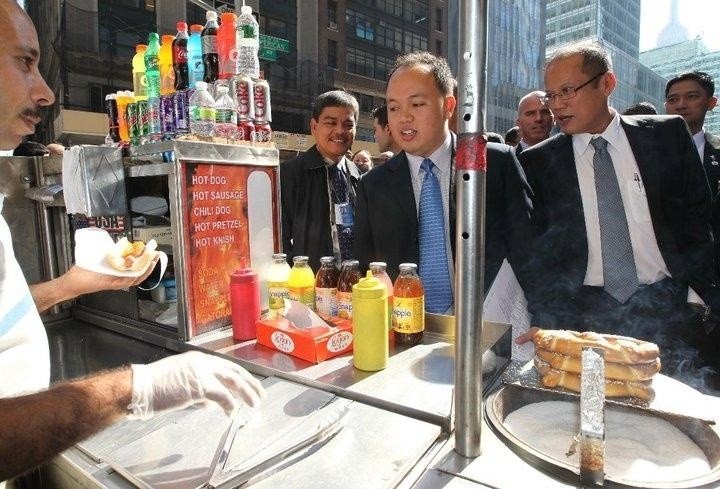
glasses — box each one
[539,73,601,110]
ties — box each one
[419,159,453,314]
[329,164,355,260]
[589,137,638,305]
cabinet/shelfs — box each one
[34,137,291,344]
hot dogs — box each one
[106,236,158,272]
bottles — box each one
[367,261,399,339]
[228,254,260,341]
[131,5,261,100]
[184,78,236,139]
[265,252,362,322]
[103,89,134,148]
[351,270,389,372]
[394,263,427,346]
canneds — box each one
[125,79,273,162]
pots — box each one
[485,381,720,487]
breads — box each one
[534,329,663,402]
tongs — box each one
[200,401,354,489]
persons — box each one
[0,0,268,485]
[14,136,65,156]
[504,38,720,373]
[281,90,362,273]
[355,50,536,319]
[345,105,403,171]
